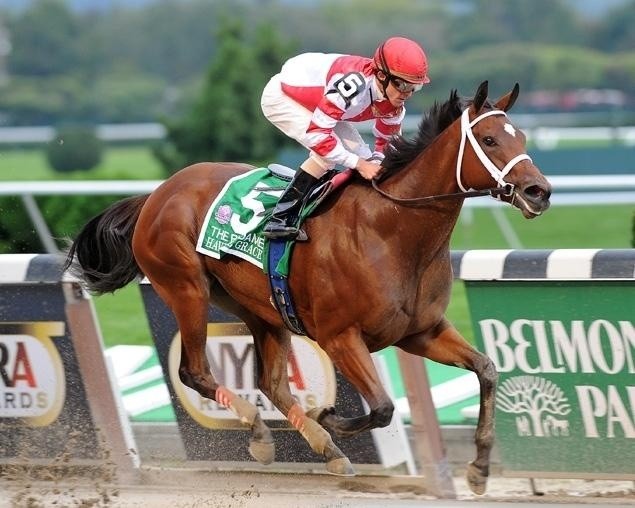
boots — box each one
[261,165,321,240]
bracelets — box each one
[342,153,360,170]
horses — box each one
[54,79,553,495]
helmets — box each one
[372,35,431,84]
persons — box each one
[261,37,432,244]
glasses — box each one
[389,76,423,94]
